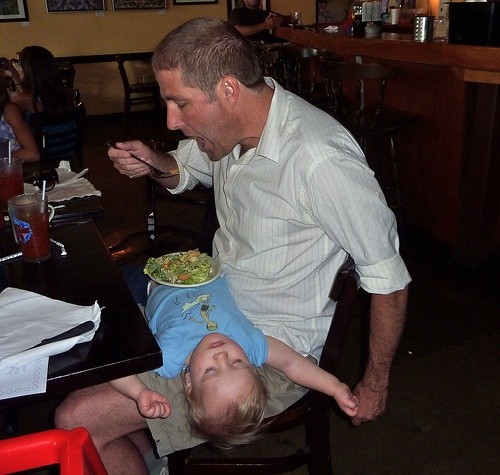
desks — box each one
[0,160,105,228]
[0,215,163,409]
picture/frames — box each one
[227,0,270,22]
[112,0,166,11]
[45,0,105,13]
[0,0,30,22]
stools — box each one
[253,41,421,226]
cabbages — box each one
[143,249,214,285]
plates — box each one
[148,252,220,288]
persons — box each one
[108,264,359,450]
[52,17,412,475]
[231,0,288,51]
[0,46,79,168]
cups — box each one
[290,8,301,24]
[391,9,400,25]
[0,157,24,213]
[7,192,52,264]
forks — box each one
[106,141,179,178]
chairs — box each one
[116,55,162,122]
[31,70,86,169]
[168,257,361,475]
[109,139,219,267]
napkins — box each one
[44,160,102,202]
[0,287,105,372]
